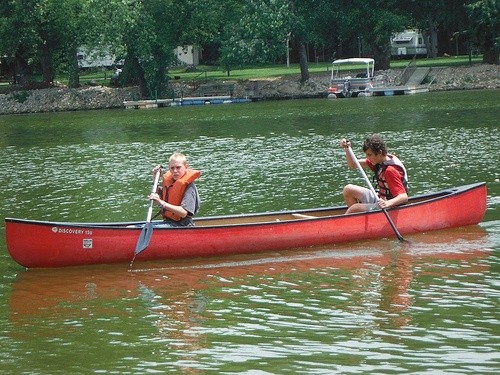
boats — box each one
[5,181,486,268]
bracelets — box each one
[159,201,166,209]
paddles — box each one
[135,164,163,254]
[345,139,409,243]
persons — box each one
[111,65,123,79]
[123,154,203,228]
[338,135,410,215]
